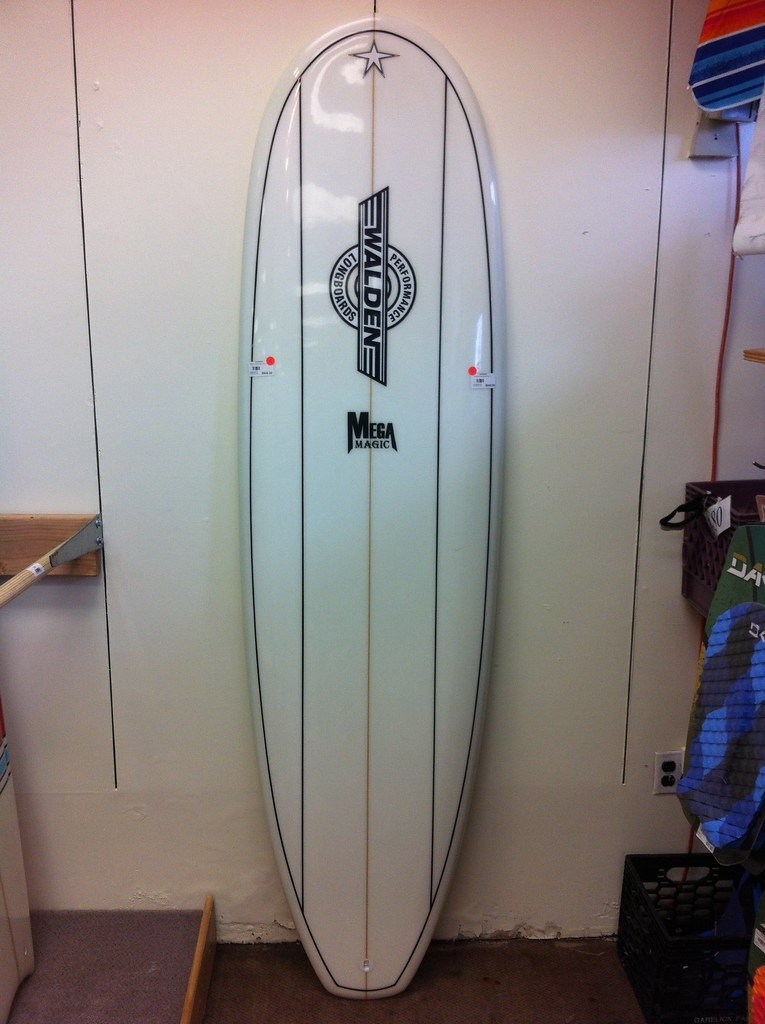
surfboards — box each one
[236,16,511,1000]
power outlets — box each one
[653,750,684,794]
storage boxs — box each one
[682,479,765,619]
[617,854,758,1024]
